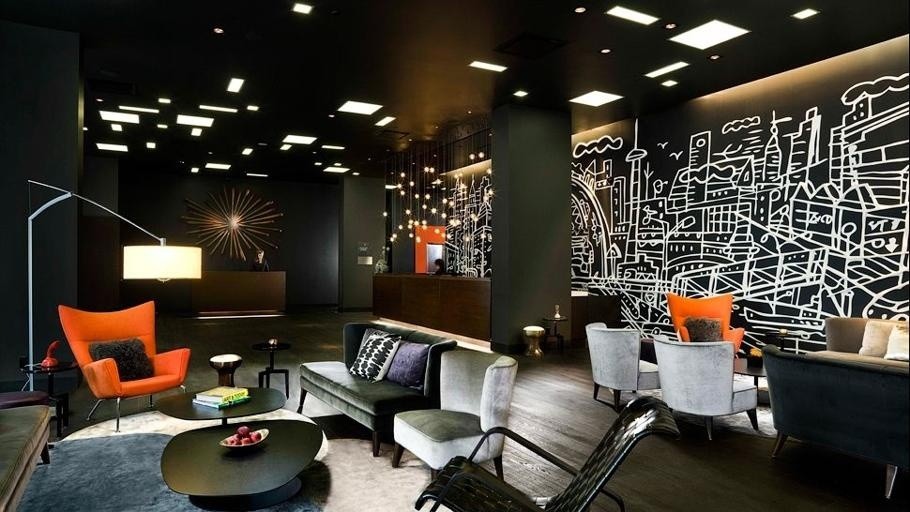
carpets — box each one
[608,372,804,444]
[17,432,331,512]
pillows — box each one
[385,340,432,391]
[884,324,909,361]
[88,337,155,382]
[349,327,402,384]
[684,316,722,342]
[858,319,904,357]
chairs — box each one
[415,395,682,512]
[585,322,661,412]
[653,334,758,441]
[667,292,745,358]
[57,300,192,433]
[392,349,519,481]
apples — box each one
[226,426,262,445]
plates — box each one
[219,427,270,452]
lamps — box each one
[20,178,203,391]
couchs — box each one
[805,316,909,374]
[0,391,52,512]
[297,322,457,457]
[761,345,910,499]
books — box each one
[196,386,248,403]
[192,396,251,409]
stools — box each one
[542,315,568,349]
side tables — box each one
[18,360,79,437]
[251,341,290,401]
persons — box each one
[254,250,269,272]
[433,259,445,275]
